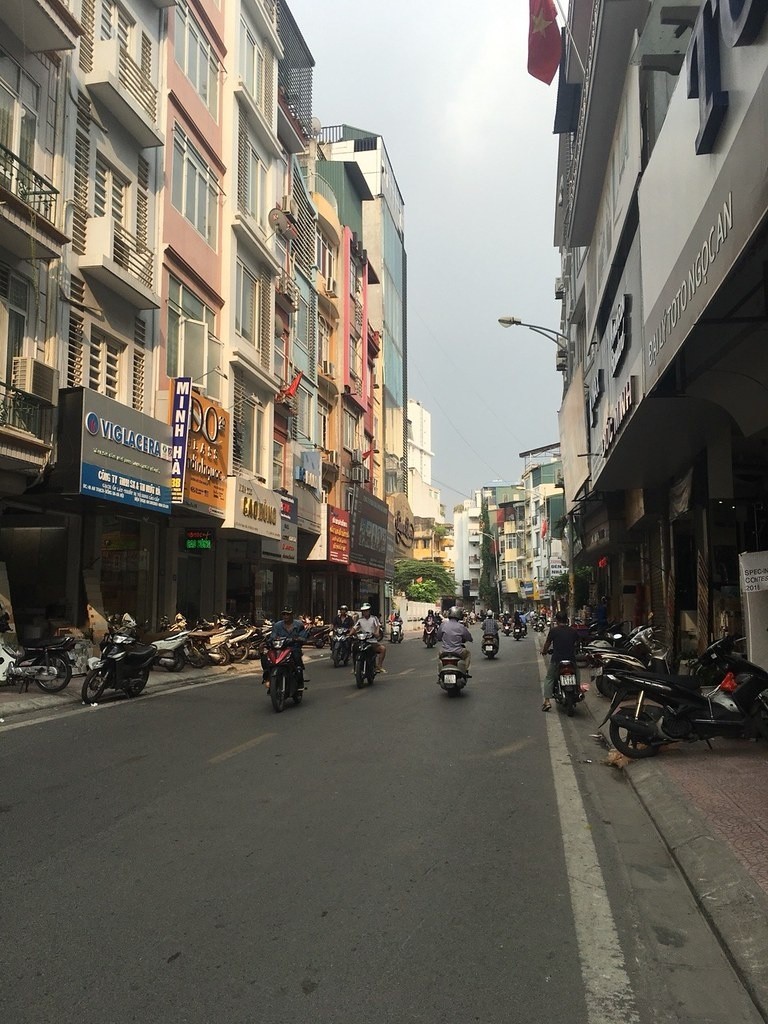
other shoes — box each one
[297,687,304,691]
[375,668,386,673]
[466,674,472,678]
[542,703,551,710]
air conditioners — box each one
[374,374,379,387]
[328,450,339,464]
[281,194,298,222]
[325,277,340,298]
[351,449,362,464]
[556,338,568,357]
[322,360,336,380]
[526,517,531,527]
[359,469,362,483]
[364,469,370,483]
[352,468,359,481]
[554,277,563,299]
[556,356,567,371]
[10,357,61,410]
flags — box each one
[285,372,302,397]
[363,450,372,461]
[541,521,547,538]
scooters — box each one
[420,616,443,649]
[115,614,276,672]
[479,626,502,659]
[261,633,310,712]
[436,639,474,698]
[304,621,385,689]
[458,618,477,628]
[499,613,547,641]
[386,617,405,643]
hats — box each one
[280,605,293,613]
[361,602,371,611]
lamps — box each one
[248,475,266,485]
[192,365,228,382]
[300,443,317,448]
[498,316,568,350]
[296,437,310,442]
[306,447,324,451]
[312,450,330,455]
[224,392,263,410]
[271,486,289,496]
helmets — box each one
[340,605,348,612]
[487,609,493,616]
[448,606,462,619]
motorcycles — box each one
[571,604,768,758]
[0,601,76,692]
[81,615,160,704]
[540,648,587,716]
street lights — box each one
[475,530,501,614]
[514,486,554,628]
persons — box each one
[436,606,473,670]
[540,611,581,711]
[530,606,547,622]
[461,608,476,622]
[391,613,403,629]
[330,605,359,654]
[260,606,308,687]
[481,609,499,645]
[423,609,449,637]
[499,610,531,634]
[596,595,610,639]
[347,603,388,673]
